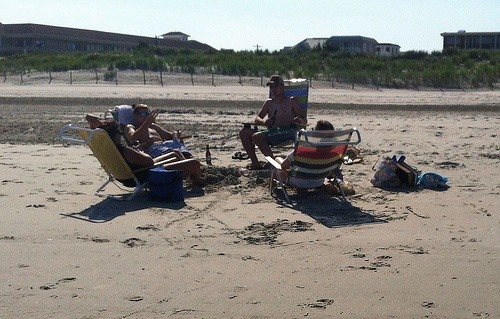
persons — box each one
[270,120,341,189]
[238,76,308,170]
[86,103,212,188]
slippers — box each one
[342,154,359,161]
[239,153,250,160]
[343,158,362,164]
[232,151,242,159]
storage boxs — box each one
[149,168,184,202]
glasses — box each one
[269,85,281,88]
[112,128,123,134]
[136,111,149,115]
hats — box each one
[97,118,120,130]
[266,75,284,86]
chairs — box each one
[58,105,190,202]
[265,126,362,205]
[242,79,308,157]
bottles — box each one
[206,143,211,163]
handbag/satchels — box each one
[369,155,407,188]
